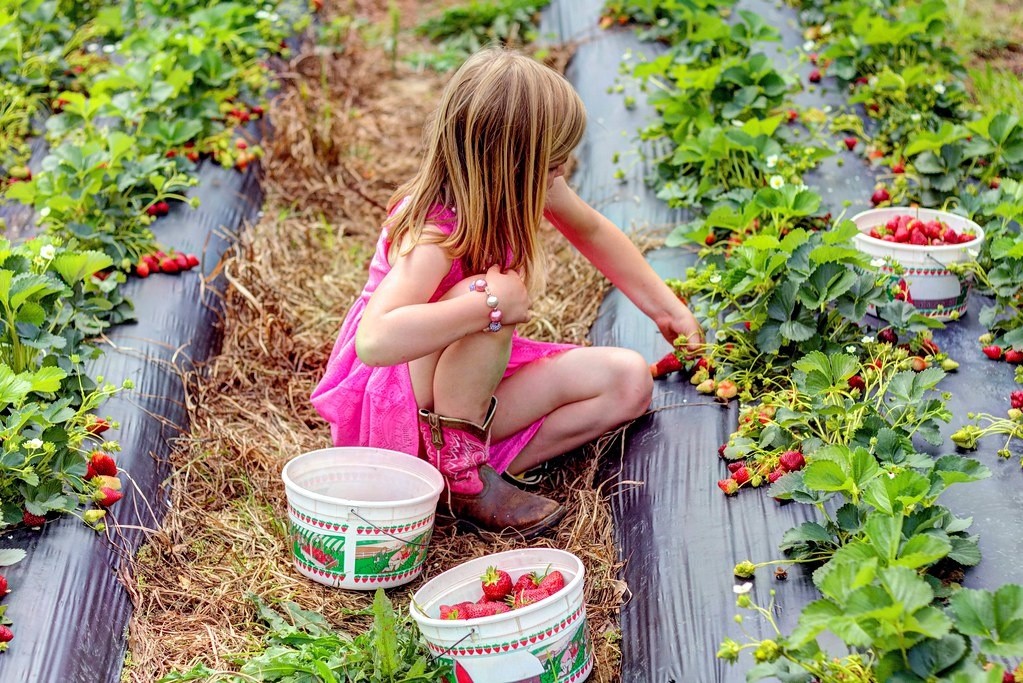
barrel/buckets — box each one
[280,446,446,592]
[847,206,985,322]
[409,547,594,683]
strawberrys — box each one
[0,0,324,642]
[600,14,1023,683]
[439,563,565,621]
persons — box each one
[308,49,706,538]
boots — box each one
[419,393,571,541]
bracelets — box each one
[468,277,504,333]
